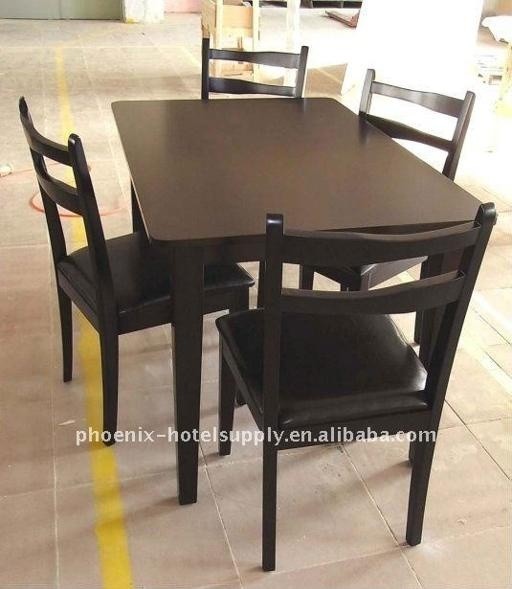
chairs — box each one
[19,36,496,572]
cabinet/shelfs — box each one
[201,0,258,82]
